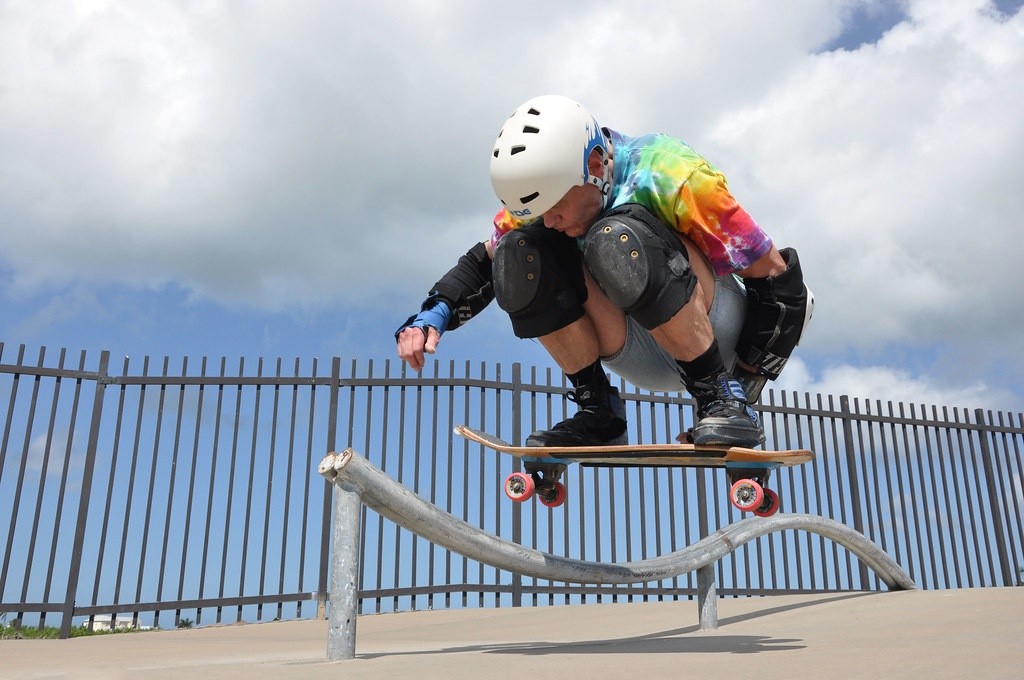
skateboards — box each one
[452,421,815,518]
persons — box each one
[393,95,808,447]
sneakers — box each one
[677,368,766,447]
[525,387,630,447]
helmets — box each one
[490,95,609,220]
